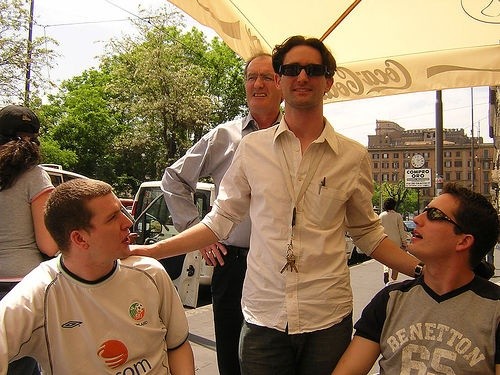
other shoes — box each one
[384,272,389,284]
[391,269,398,280]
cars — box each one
[403,220,416,232]
[38,162,217,309]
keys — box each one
[279,253,298,274]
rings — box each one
[207,251,211,254]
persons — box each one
[0,179,194,375]
[378,198,408,285]
[331,181,500,375]
[161,54,284,375]
[0,105,60,375]
[121,35,425,375]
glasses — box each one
[279,64,326,77]
[421,206,467,234]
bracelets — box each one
[414,262,425,279]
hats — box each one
[0,105,40,137]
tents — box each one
[164,0,500,105]
